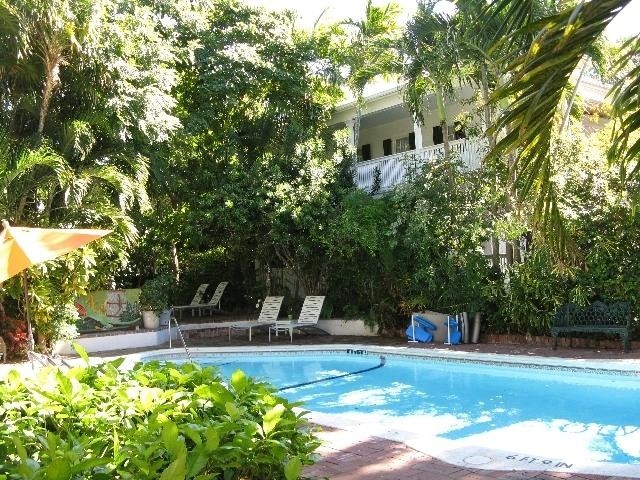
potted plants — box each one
[138,280,165,330]
[159,277,177,327]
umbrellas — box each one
[1,219,114,373]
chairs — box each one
[551,303,632,353]
[170,283,209,317]
[268,296,330,344]
[170,281,230,321]
[229,296,285,342]
